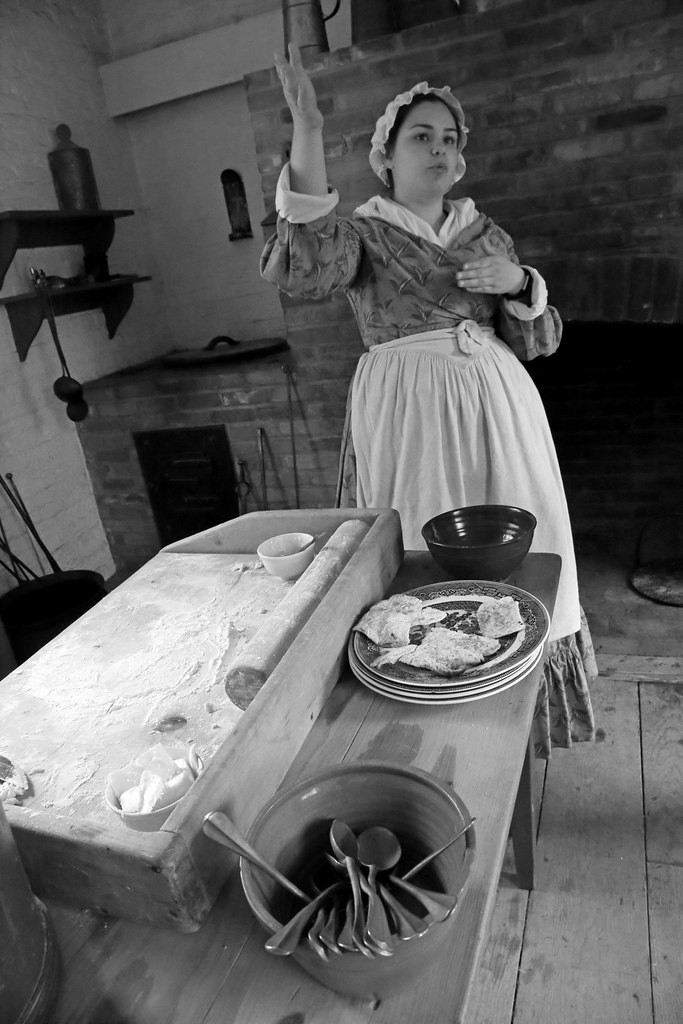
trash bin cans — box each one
[0,569,107,663]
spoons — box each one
[329,818,375,962]
[357,824,403,950]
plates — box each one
[347,580,550,704]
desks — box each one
[36,547,564,1024]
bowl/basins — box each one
[256,533,315,579]
[104,748,203,831]
[421,504,537,583]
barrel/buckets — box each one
[0,570,110,666]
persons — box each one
[256,40,597,754]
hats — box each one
[369,81,470,188]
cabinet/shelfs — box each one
[0,208,152,362]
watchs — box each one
[504,266,532,301]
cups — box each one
[239,759,476,1001]
